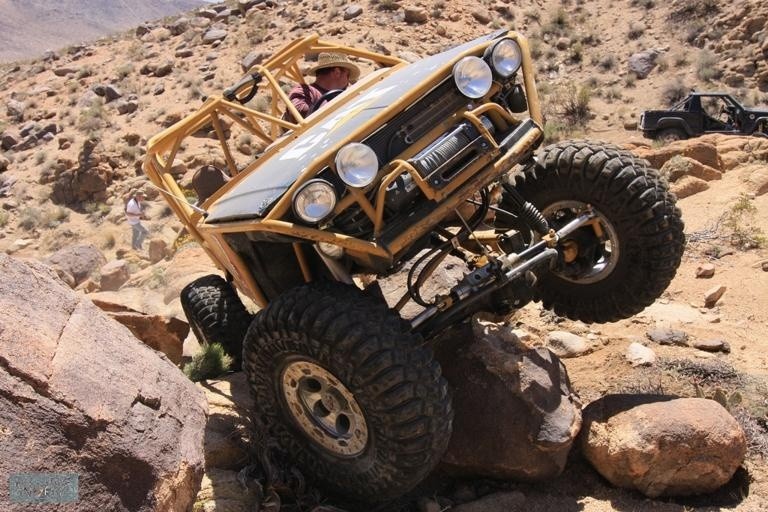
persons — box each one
[284,50,359,131]
[124,192,149,251]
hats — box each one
[133,189,147,198]
[307,52,360,80]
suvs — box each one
[141,30,688,509]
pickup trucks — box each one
[637,90,768,142]
